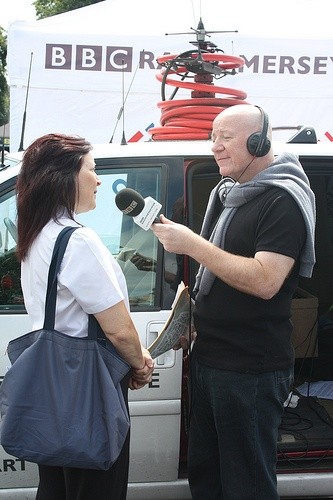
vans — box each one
[1,19,333,500]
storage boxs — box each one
[289,287,319,359]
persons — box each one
[152,104,308,500]
[15,133,152,499]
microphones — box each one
[115,188,164,231]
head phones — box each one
[247,105,271,157]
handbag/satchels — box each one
[0,225,133,471]
